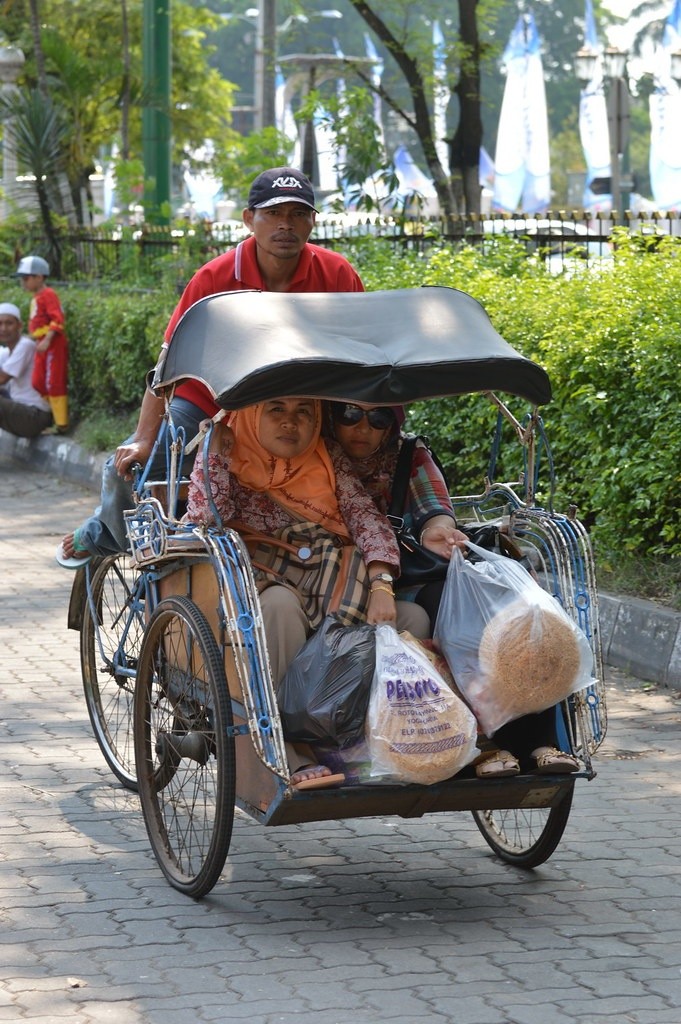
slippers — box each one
[54,527,92,568]
[292,763,345,789]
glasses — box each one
[329,402,395,431]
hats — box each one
[10,255,50,278]
[0,302,20,318]
[248,167,319,214]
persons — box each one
[0,255,69,439]
[186,398,582,791]
[55,167,365,569]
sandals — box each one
[478,749,520,776]
[527,747,580,777]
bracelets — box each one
[370,587,396,597]
[419,527,430,546]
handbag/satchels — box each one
[392,526,497,589]
[432,540,600,740]
[276,610,481,787]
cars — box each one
[212,211,608,271]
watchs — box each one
[369,572,394,586]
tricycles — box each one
[66,283,604,897]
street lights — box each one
[572,46,632,224]
